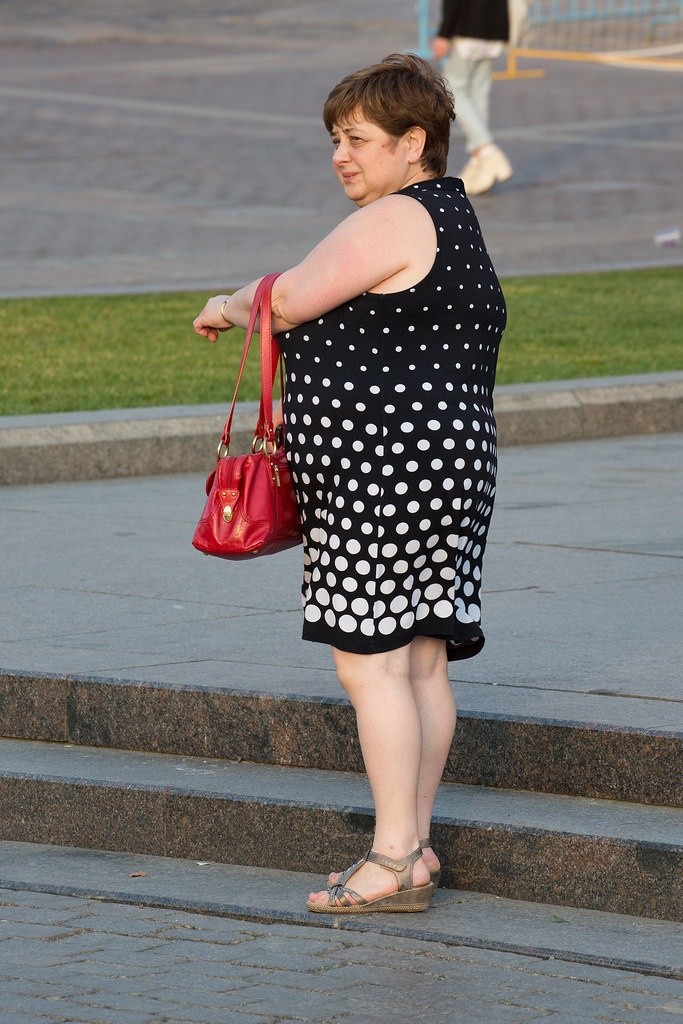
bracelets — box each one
[220,295,235,328]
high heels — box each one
[460,147,513,195]
[326,837,442,896]
[305,847,435,913]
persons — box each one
[426,0,516,198]
[193,52,507,914]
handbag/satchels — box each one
[191,271,303,559]
